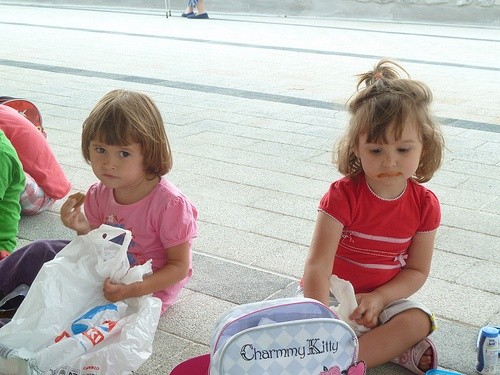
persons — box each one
[0,88,198,331]
[0,128,26,259]
[181,0,209,19]
[0,95,73,213]
[302,59,442,375]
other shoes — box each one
[187,13,209,19]
[182,11,195,17]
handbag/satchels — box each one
[207,298,367,375]
[0,224,162,375]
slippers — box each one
[391,338,438,375]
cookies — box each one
[68,192,86,208]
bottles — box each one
[476,326,500,375]
